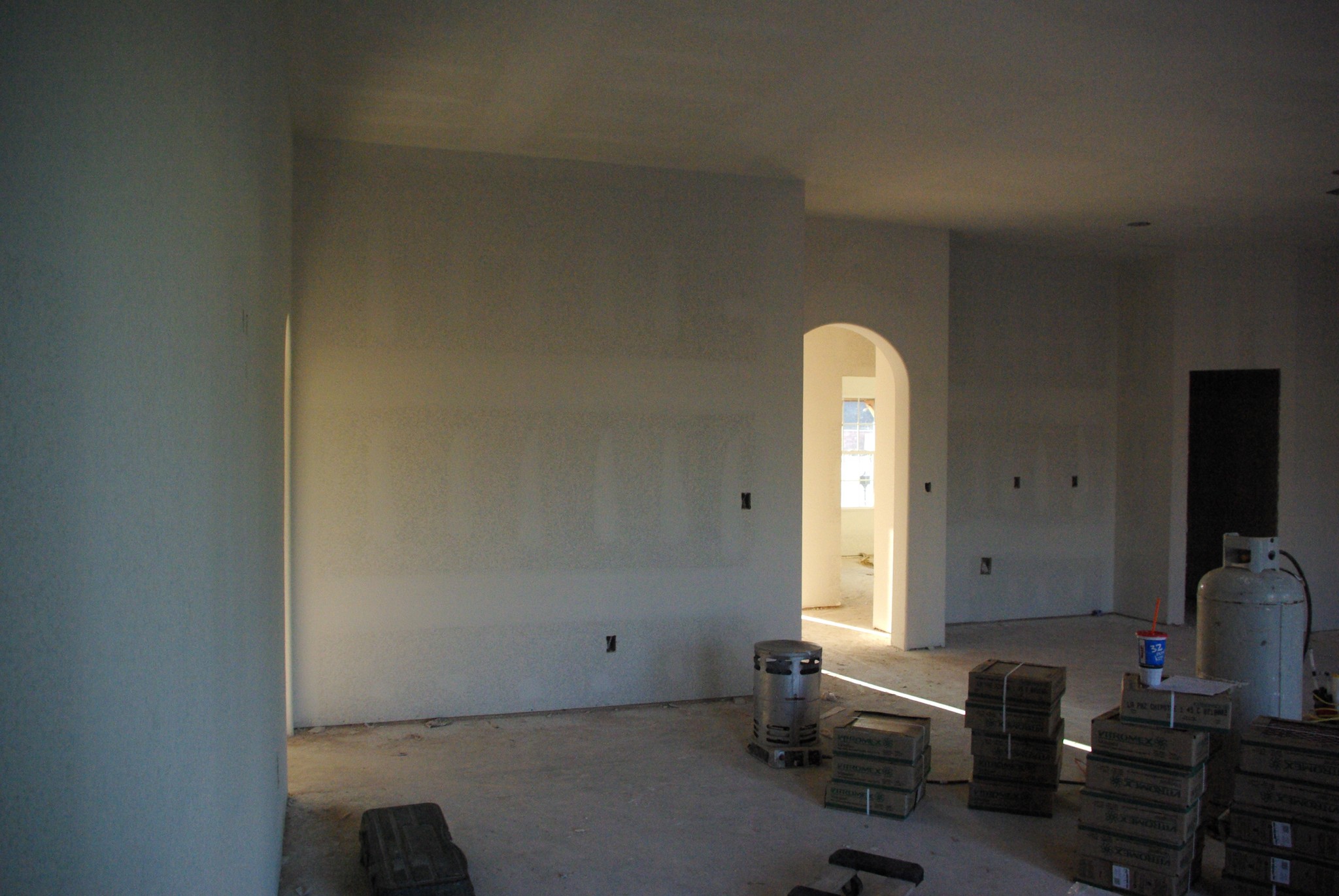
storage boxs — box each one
[358,802,476,896]
[963,658,1068,820]
[823,708,932,823]
[1072,670,1339,896]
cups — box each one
[1135,630,1168,686]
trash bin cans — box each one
[749,638,827,768]
